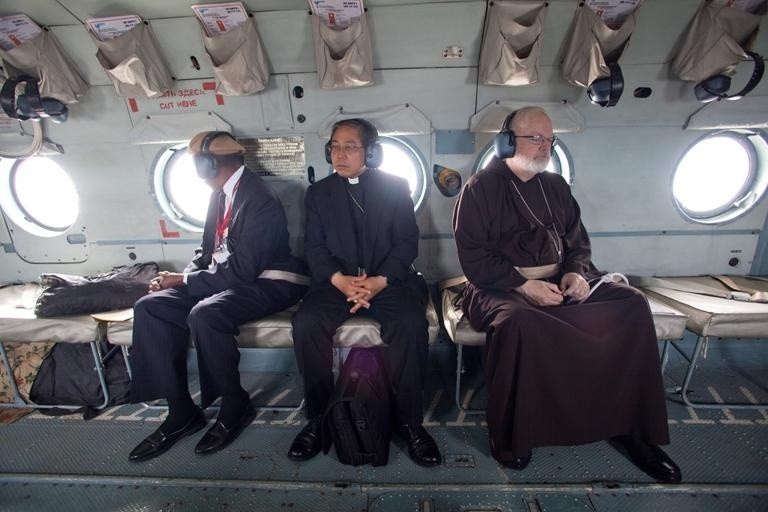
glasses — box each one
[327,143,363,153]
[515,134,558,148]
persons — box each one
[286,117,441,466]
[452,109,682,484]
[128,131,310,463]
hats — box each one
[189,131,247,158]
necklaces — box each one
[508,174,566,263]
[345,185,366,216]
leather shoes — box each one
[488,433,531,471]
[396,414,441,467]
[619,433,681,484]
[129,404,205,461]
[195,394,255,455]
[289,417,326,460]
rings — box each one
[353,300,357,303]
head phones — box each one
[325,118,383,169]
[0,75,69,124]
[495,109,517,160]
[195,131,235,179]
[586,57,625,109]
[694,50,765,104]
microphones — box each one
[681,103,709,131]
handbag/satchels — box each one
[307,8,374,89]
[326,347,392,464]
[89,21,170,98]
[0,26,88,121]
[560,1,643,89]
[479,0,548,87]
[3,341,54,424]
[30,343,132,419]
[670,0,768,81]
[35,262,158,316]
[197,17,269,96]
